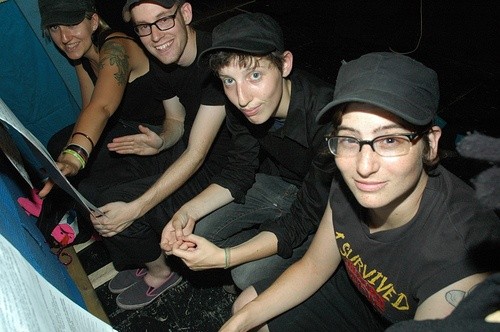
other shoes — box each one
[222,276,242,294]
[117,269,183,310]
[109,266,148,292]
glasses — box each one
[323,128,426,157]
[132,4,183,37]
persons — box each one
[90,0,234,309]
[161,12,336,291]
[38,0,166,221]
[220,53,500,332]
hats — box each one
[196,13,289,69]
[123,0,176,23]
[38,0,91,32]
[316,56,443,136]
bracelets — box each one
[225,247,230,270]
[63,143,89,169]
[72,132,94,150]
